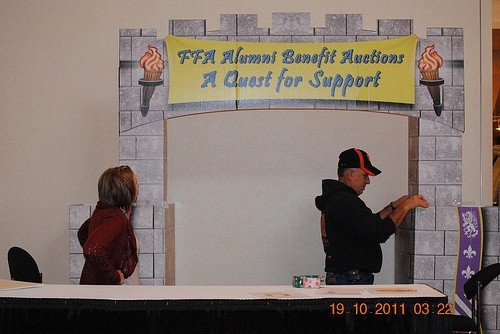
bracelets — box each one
[390,201,396,211]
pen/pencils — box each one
[376,288,418,292]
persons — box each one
[315,147,429,285]
[75,165,140,285]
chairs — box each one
[8,246,42,284]
[450,263,500,334]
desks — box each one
[0,280,450,334]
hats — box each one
[338,148,382,176]
